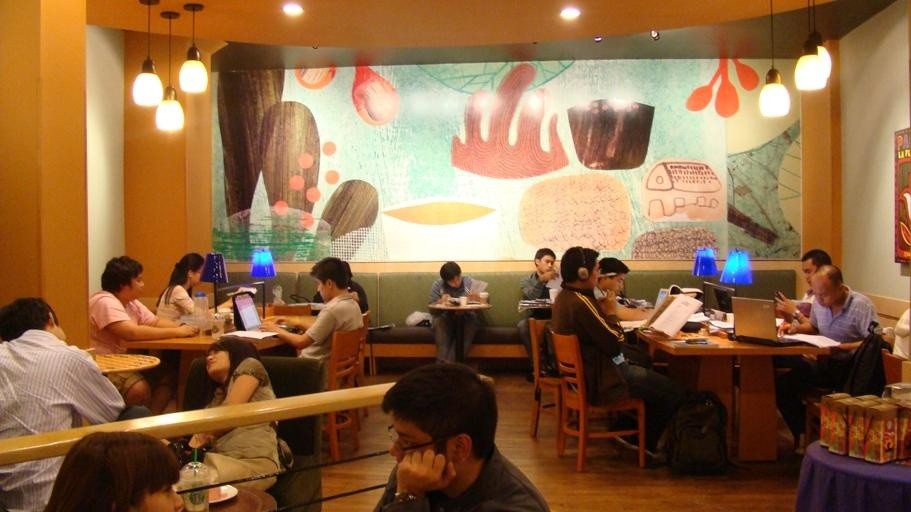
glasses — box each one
[384,423,459,454]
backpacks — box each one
[663,388,731,477]
[836,319,895,401]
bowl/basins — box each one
[681,321,704,334]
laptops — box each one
[731,296,803,346]
[703,281,736,322]
[233,292,300,339]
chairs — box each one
[267,302,311,316]
[362,310,370,416]
[183,356,328,512]
[542,326,646,471]
[528,316,578,436]
[320,328,362,458]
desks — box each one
[120,325,303,412]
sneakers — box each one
[607,425,660,461]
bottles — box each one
[192,291,208,329]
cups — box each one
[460,296,468,306]
[549,288,558,304]
[179,461,212,512]
[479,291,489,306]
[209,314,235,341]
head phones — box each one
[575,246,590,281]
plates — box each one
[172,482,239,504]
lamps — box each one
[813,0,833,81]
[177,3,210,93]
[251,250,277,318]
[198,251,228,318]
[131,0,164,108]
[692,248,718,281]
[154,10,184,133]
[794,1,827,91]
[757,0,790,118]
[719,249,752,297]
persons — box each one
[2,247,371,511]
[374,363,549,510]
[514,247,909,465]
[426,260,486,364]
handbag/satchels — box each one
[275,432,299,475]
